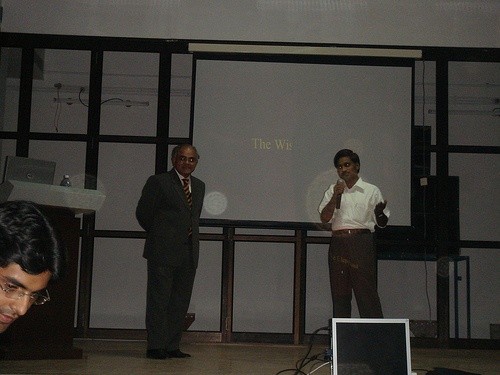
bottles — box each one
[61,174,72,186]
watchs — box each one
[378,214,385,219]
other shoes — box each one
[143,347,192,358]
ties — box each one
[181,178,193,238]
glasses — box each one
[1,285,51,306]
[336,161,352,169]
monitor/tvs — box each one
[328,317,411,375]
[2,156,56,185]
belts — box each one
[332,228,369,235]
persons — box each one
[135,142,207,359]
[0,201,58,334]
[317,149,390,319]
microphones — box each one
[336,179,345,209]
